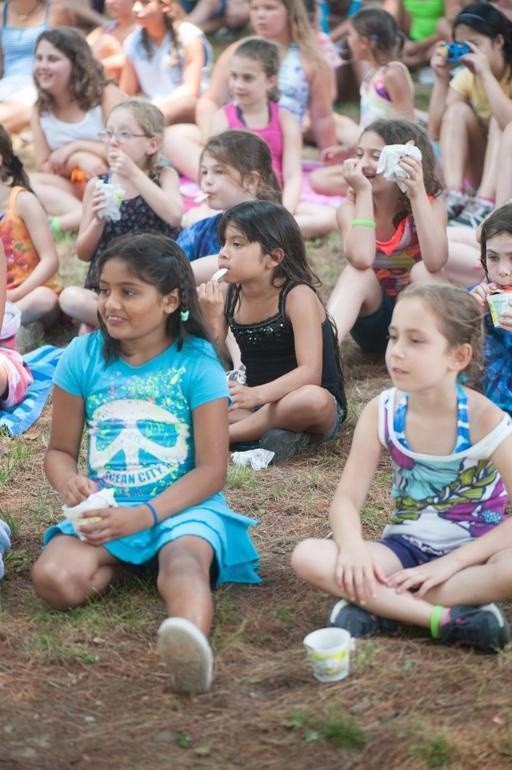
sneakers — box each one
[152,613,218,696]
[439,184,496,228]
[436,602,510,654]
[256,426,315,464]
[325,595,402,641]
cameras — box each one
[444,43,468,62]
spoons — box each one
[212,268,228,281]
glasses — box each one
[97,127,153,144]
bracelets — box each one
[140,500,159,531]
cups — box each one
[303,627,351,684]
[63,498,103,542]
[487,293,511,330]
[382,144,410,183]
[98,184,125,216]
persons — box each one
[0,0,512,467]
[29,232,233,697]
[291,280,512,654]
